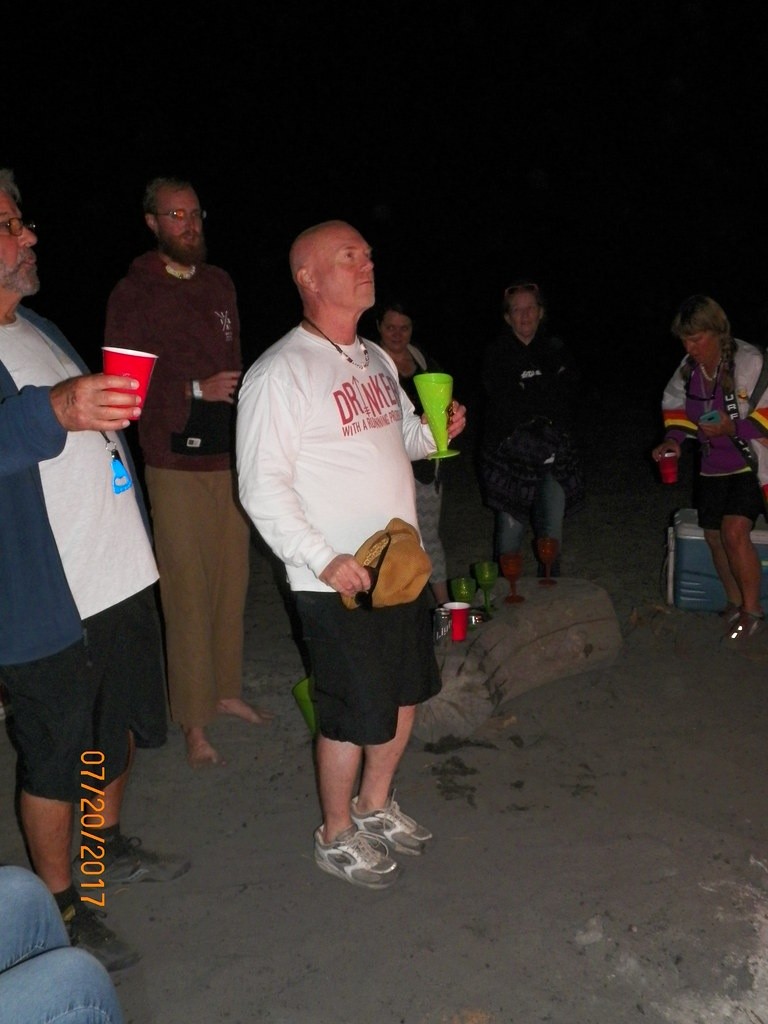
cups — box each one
[443,602,471,641]
[451,578,476,608]
[101,346,159,421]
[658,452,678,483]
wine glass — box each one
[499,552,525,604]
[413,372,460,459]
[474,561,499,613]
[538,537,559,587]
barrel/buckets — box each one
[292,676,319,735]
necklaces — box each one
[164,265,200,281]
[299,311,372,371]
[698,362,722,381]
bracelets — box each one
[192,379,200,401]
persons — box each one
[482,281,570,579]
[104,177,280,771]
[1,175,194,972]
[235,219,468,890]
[1,866,132,1024]
[376,302,451,605]
[650,295,768,652]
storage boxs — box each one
[665,508,768,618]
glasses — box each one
[504,283,540,307]
[147,209,207,221]
[0,217,36,236]
[685,365,720,401]
[355,532,392,612]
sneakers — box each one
[313,822,401,889]
[62,894,143,972]
[71,833,191,892]
[350,788,433,857]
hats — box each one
[341,518,433,610]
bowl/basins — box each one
[466,609,490,625]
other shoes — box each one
[719,603,742,635]
[721,612,767,649]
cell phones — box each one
[700,412,722,425]
